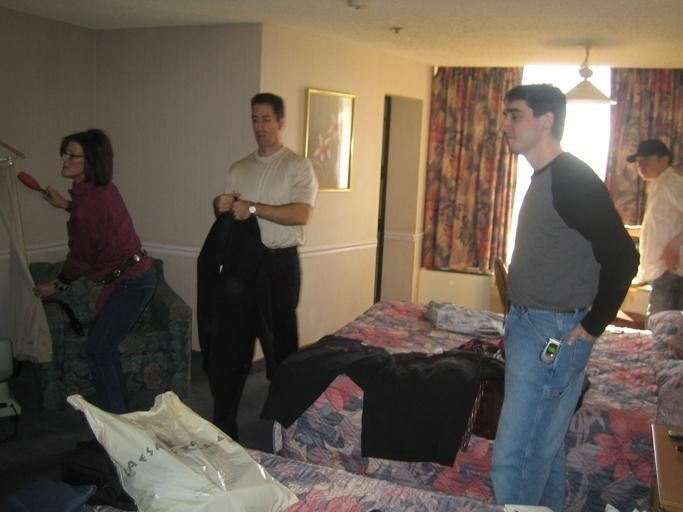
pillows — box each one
[653,359,683,425]
[648,310,683,364]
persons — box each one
[626,139,683,330]
[32,129,159,414]
[211,93,320,420]
[487,82,640,512]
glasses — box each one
[62,150,85,160]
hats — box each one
[626,140,670,162]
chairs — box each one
[29,258,192,415]
[493,260,508,307]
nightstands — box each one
[650,423,683,512]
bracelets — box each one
[52,279,68,293]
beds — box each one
[272,299,658,512]
[77,448,520,512]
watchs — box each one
[247,202,257,220]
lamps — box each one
[565,47,617,105]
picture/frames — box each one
[302,88,357,192]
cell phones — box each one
[668,430,683,439]
[541,335,564,364]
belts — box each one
[100,249,145,284]
